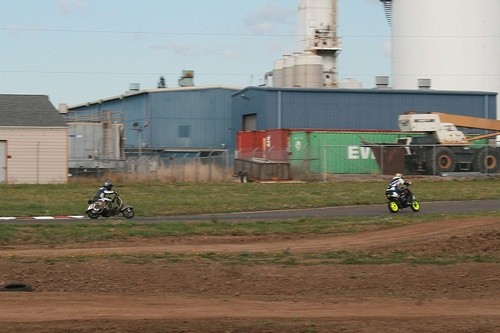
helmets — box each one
[393,173,403,179]
[103,181,113,188]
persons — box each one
[94,179,116,209]
[386,173,411,205]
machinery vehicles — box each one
[397,111,500,174]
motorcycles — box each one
[84,190,134,219]
[386,183,419,213]
